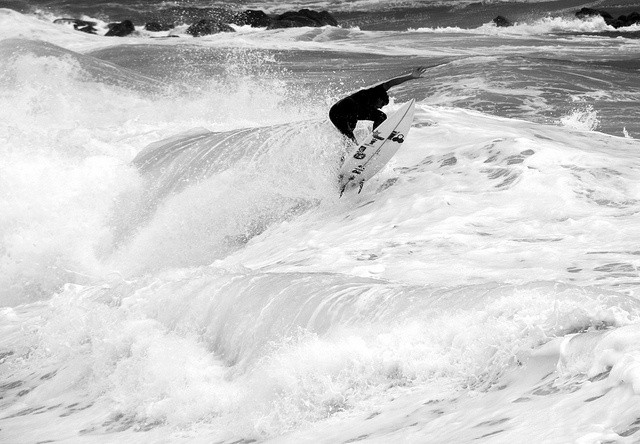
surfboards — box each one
[339,98,415,198]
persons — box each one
[329,65,426,146]
